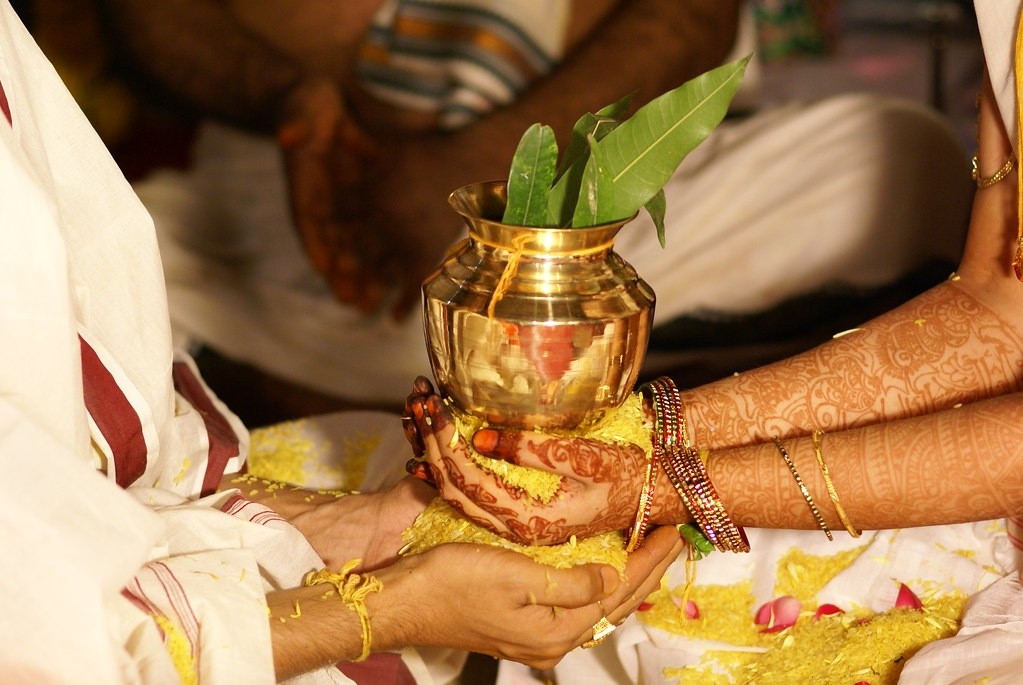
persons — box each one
[0,0,683,685]
[24,0,974,405]
[403,0,1023,685]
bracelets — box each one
[660,448,751,553]
[625,451,660,553]
[647,376,683,450]
[810,428,862,539]
[289,558,383,664]
[772,435,843,541]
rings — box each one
[580,600,618,649]
